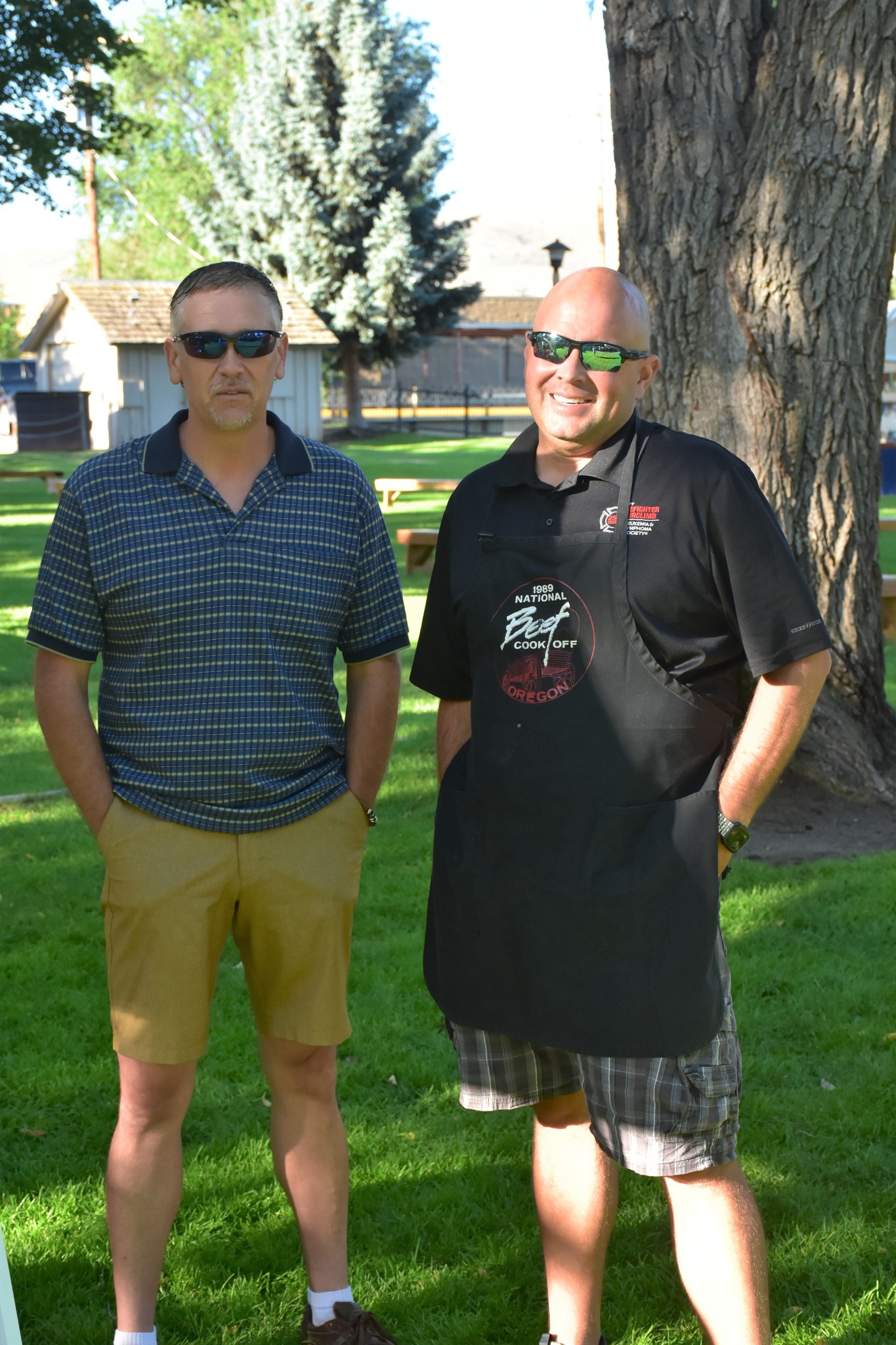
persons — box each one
[28,260,414,1345]
[405,266,835,1345]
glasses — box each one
[525,330,651,373]
[167,330,282,359]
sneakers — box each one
[299,1301,398,1345]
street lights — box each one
[543,239,572,286]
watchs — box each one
[712,814,753,856]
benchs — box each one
[396,528,439,571]
[374,477,462,507]
[0,467,62,481]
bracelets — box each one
[359,799,378,827]
